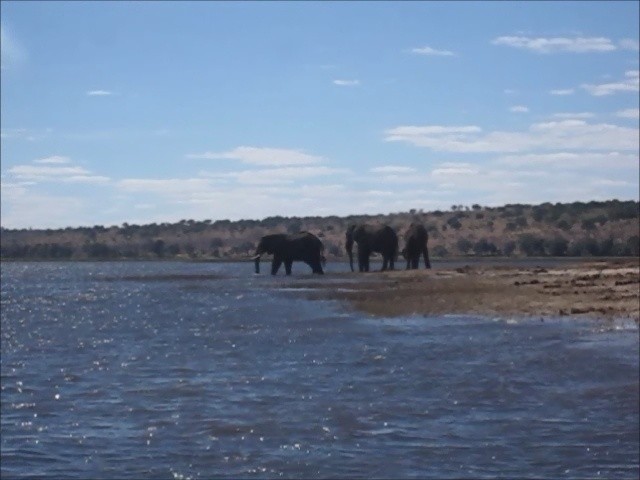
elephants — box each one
[345,222,399,272]
[248,230,326,276]
[401,223,432,270]
